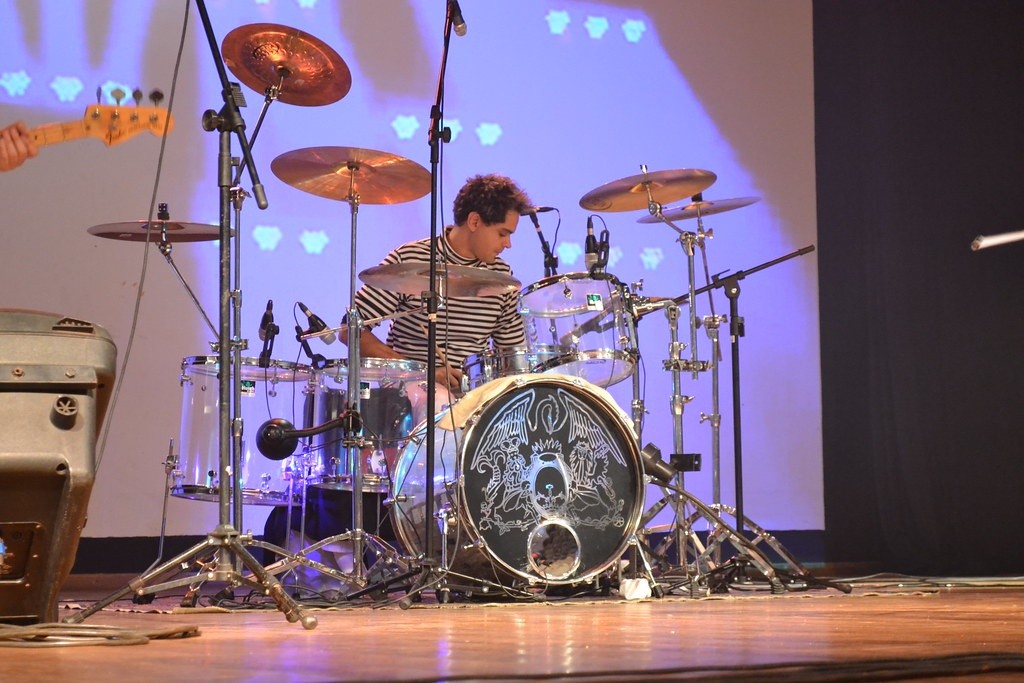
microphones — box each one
[520,206,555,216]
[449,0,467,36]
[585,218,600,271]
[259,300,274,340]
[297,302,336,345]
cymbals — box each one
[635,196,761,225]
[220,22,353,109]
[578,168,718,213]
[86,219,235,243]
[269,145,433,205]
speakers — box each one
[0,305,117,626]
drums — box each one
[515,271,638,387]
[169,355,314,508]
[463,342,579,394]
[387,375,648,587]
[301,357,430,494]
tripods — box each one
[639,184,852,595]
[62,1,548,629]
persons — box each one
[339,175,531,426]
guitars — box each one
[1,86,175,149]
